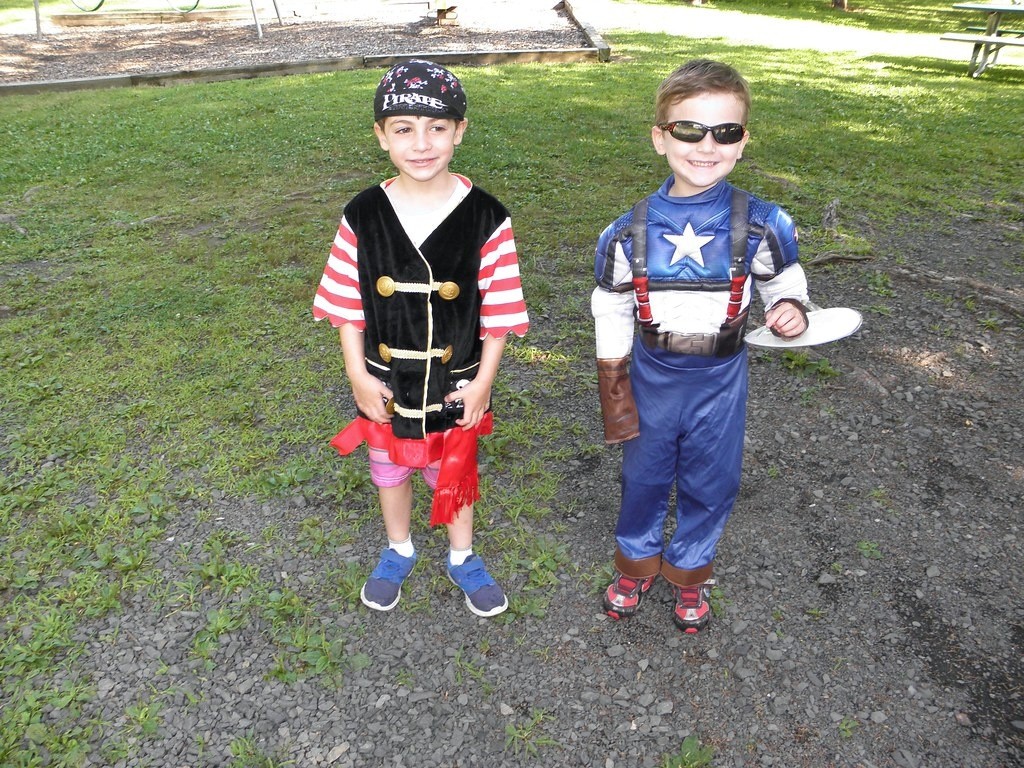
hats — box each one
[374,59,467,121]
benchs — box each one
[940,26,1024,47]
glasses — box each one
[658,120,747,144]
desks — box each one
[952,3,1024,79]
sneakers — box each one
[360,546,417,612]
[670,577,716,633]
[600,567,659,620]
[445,551,509,617]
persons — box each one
[590,57,812,639]
[310,57,531,618]
